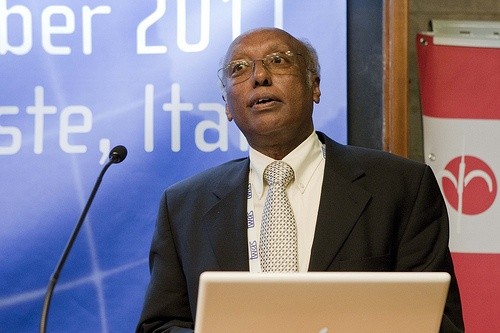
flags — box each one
[416,30,500,333]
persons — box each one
[136,28,466,333]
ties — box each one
[259,160,299,272]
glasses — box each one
[216,50,316,105]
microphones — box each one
[40,145,127,333]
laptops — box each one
[194,271,449,333]
